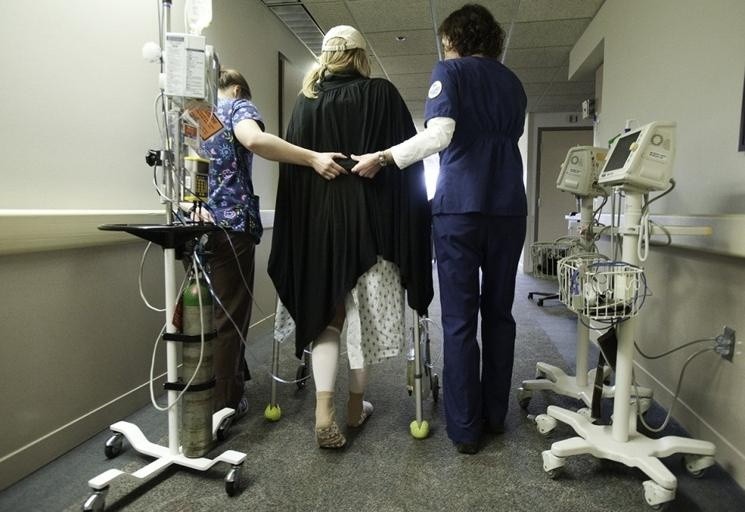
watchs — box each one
[377,150,390,169]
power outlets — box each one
[720,326,736,362]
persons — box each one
[268,24,433,455]
[190,67,350,421]
[345,3,533,457]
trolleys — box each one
[264,307,440,438]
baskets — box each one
[557,256,644,320]
[530,241,572,279]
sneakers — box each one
[229,398,249,421]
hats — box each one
[322,25,367,51]
